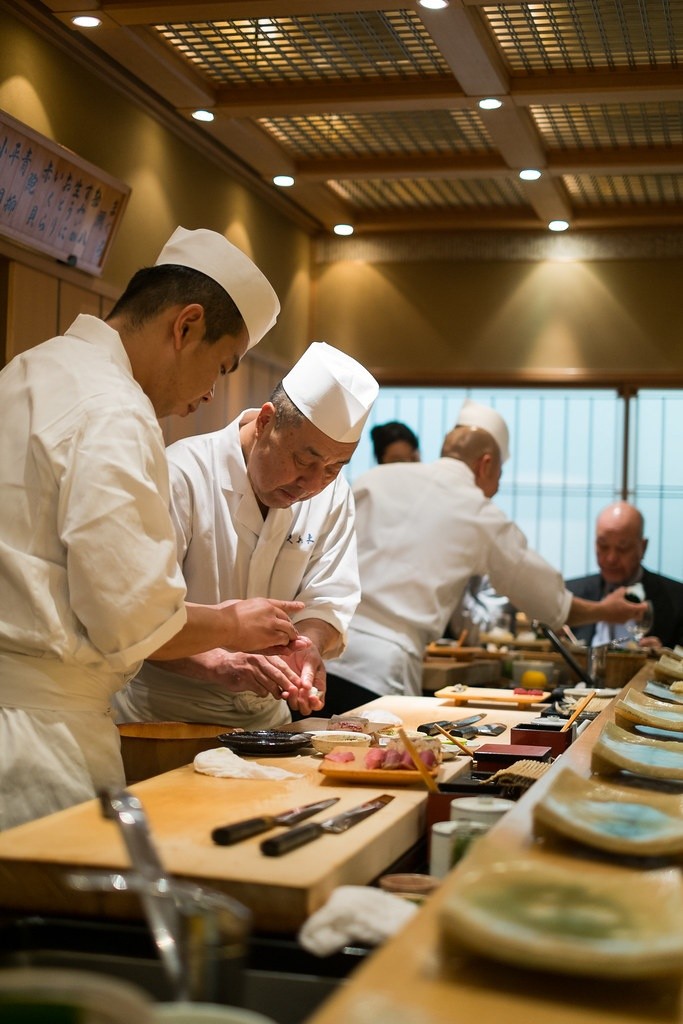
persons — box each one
[499,500,683,657]
[371,422,421,463]
[111,342,379,728]
[0,224,305,834]
[290,397,650,724]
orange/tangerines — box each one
[522,670,547,691]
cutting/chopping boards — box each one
[270,716,394,735]
[0,695,540,939]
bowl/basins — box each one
[312,735,371,756]
[374,731,427,745]
[424,736,466,761]
[448,660,502,687]
[513,661,552,690]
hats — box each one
[153,225,281,350]
[456,401,509,463]
[283,342,379,443]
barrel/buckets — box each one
[115,722,241,787]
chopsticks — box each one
[560,691,596,732]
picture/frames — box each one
[0,108,132,277]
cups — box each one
[626,600,653,640]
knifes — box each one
[417,713,487,736]
[211,798,340,846]
[449,723,507,740]
[260,794,395,857]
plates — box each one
[434,685,551,703]
[438,656,683,978]
[564,688,620,697]
[217,730,315,755]
[319,746,440,784]
[303,730,371,741]
